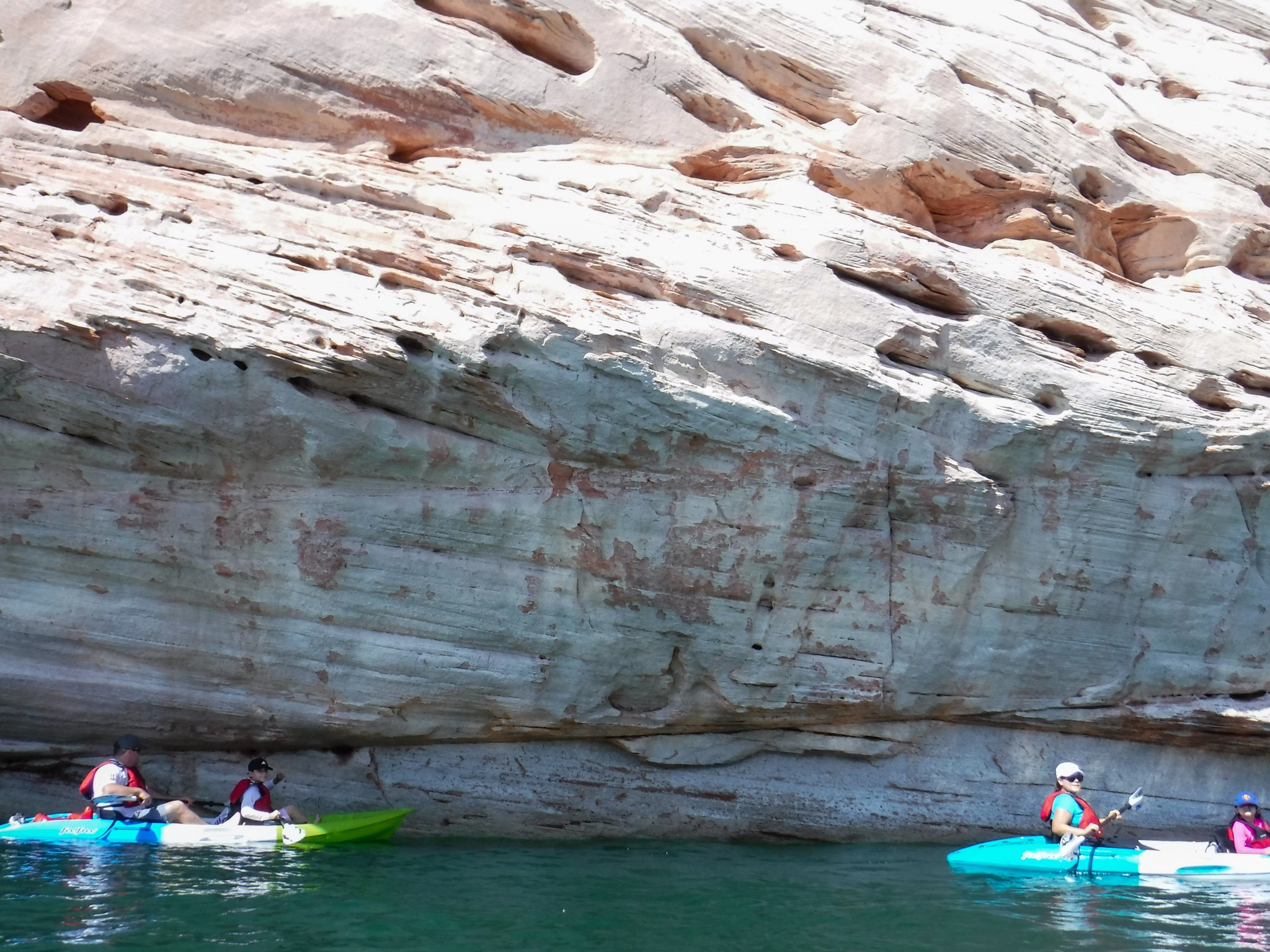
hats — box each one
[1056,762,1084,778]
[1235,791,1258,807]
[114,735,146,750]
[248,758,273,770]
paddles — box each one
[90,794,232,808]
[271,805,305,845]
[1023,786,1144,862]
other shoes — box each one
[218,811,242,826]
[211,807,231,825]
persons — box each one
[1232,791,1270,856]
[92,734,241,825]
[1050,762,1141,850]
[230,758,322,826]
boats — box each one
[946,836,1270,876]
[0,804,417,851]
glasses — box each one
[1063,775,1083,782]
[125,748,141,753]
